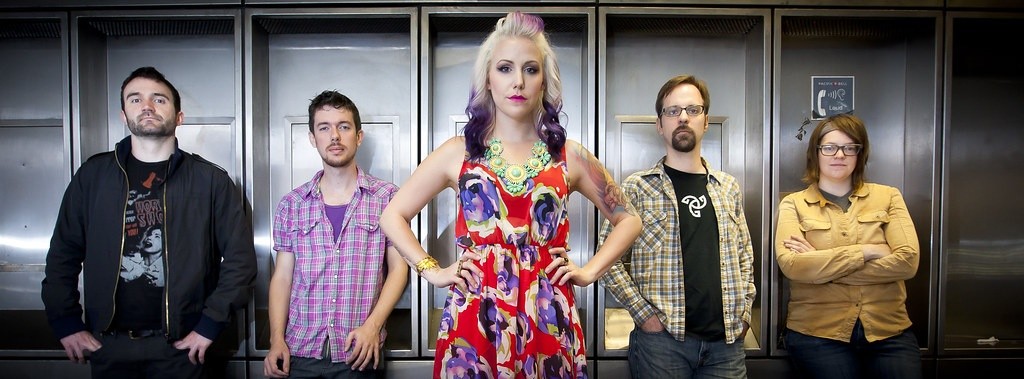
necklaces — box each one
[484,136,551,193]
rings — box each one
[565,266,569,272]
[456,259,463,275]
[563,257,569,265]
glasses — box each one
[659,104,705,116]
[816,143,864,156]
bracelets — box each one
[414,256,440,274]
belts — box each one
[103,328,162,339]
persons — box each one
[40,67,258,379]
[253,91,409,379]
[597,73,757,379]
[386,9,644,379]
[775,114,921,379]
